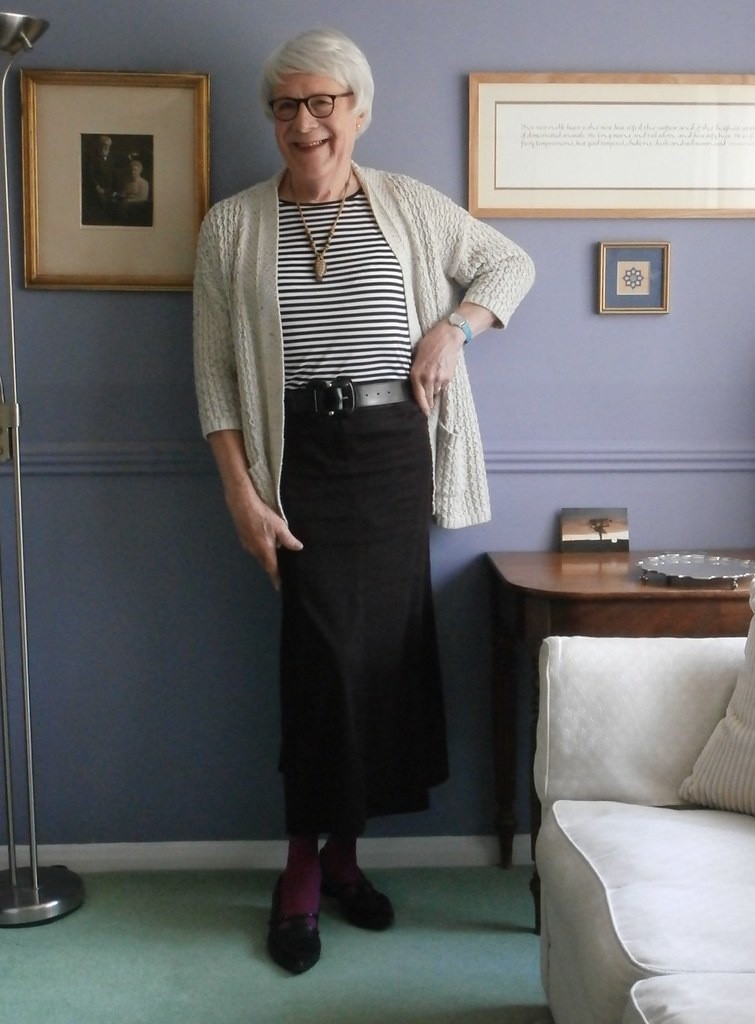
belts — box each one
[285,376,414,413]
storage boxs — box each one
[559,508,630,551]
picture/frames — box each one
[466,71,754,220]
[599,241,670,316]
[19,68,210,290]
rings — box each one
[434,386,441,395]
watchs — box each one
[443,312,474,345]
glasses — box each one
[269,92,353,121]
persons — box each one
[194,28,533,974]
[85,136,148,212]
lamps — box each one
[0,11,60,928]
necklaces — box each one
[284,168,357,280]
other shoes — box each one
[319,850,395,929]
[268,878,321,973]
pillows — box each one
[679,580,755,814]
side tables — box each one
[480,550,755,933]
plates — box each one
[636,553,755,589]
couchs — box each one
[535,635,755,1024]
